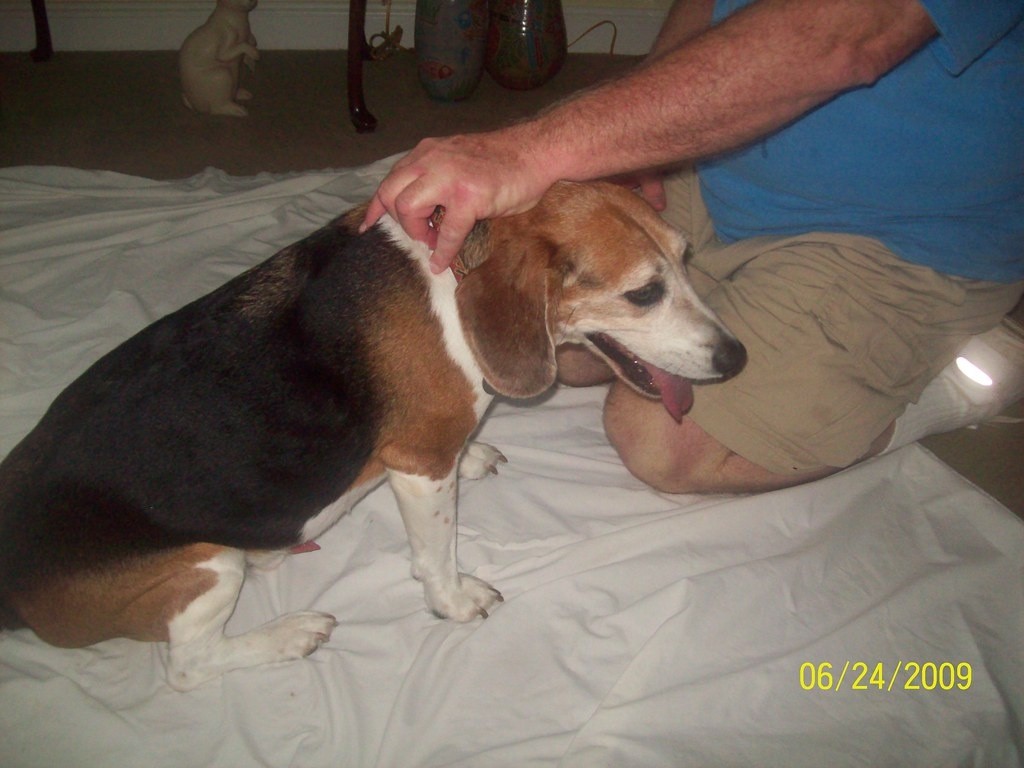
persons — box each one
[357,1,1024,496]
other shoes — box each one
[941,315,1024,422]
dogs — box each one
[0,178,747,690]
